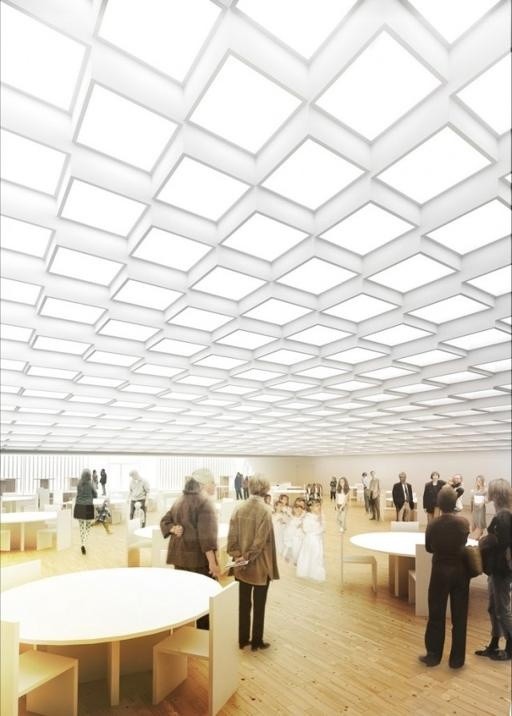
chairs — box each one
[383,491,418,522]
[350,488,367,508]
[391,521,418,532]
[152,581,240,716]
[128,529,175,569]
[0,619,78,716]
[341,531,377,593]
[408,544,451,616]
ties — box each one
[404,484,409,503]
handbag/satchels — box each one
[465,544,484,578]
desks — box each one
[1,567,223,707]
[0,487,77,514]
[72,492,184,526]
[350,532,479,598]
[134,523,229,586]
[214,498,243,523]
[0,511,57,552]
[267,487,307,508]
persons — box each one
[227,474,280,651]
[74,471,97,555]
[474,478,512,661]
[100,469,106,495]
[90,498,112,535]
[160,468,221,630]
[92,470,98,489]
[127,469,152,528]
[418,484,470,668]
[234,472,489,583]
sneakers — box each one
[250,639,271,652]
[418,655,438,666]
[474,646,512,661]
[81,545,86,555]
[239,641,250,649]
